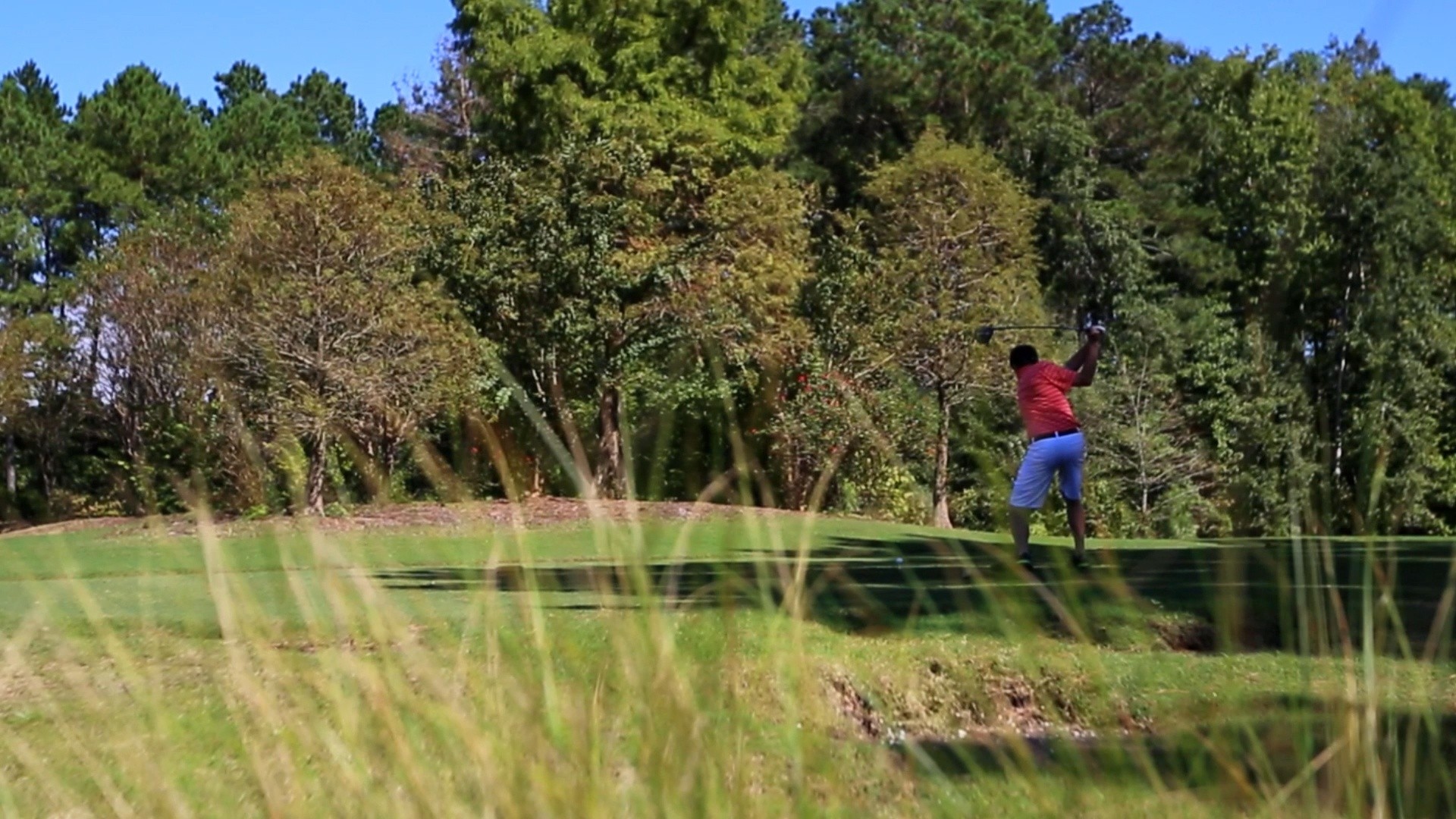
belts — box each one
[1029,427,1080,443]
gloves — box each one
[1085,318,1106,338]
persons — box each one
[1007,326,1105,568]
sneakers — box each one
[1068,554,1091,572]
[1016,552,1033,571]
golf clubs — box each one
[976,326,1090,344]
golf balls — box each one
[896,557,904,563]
